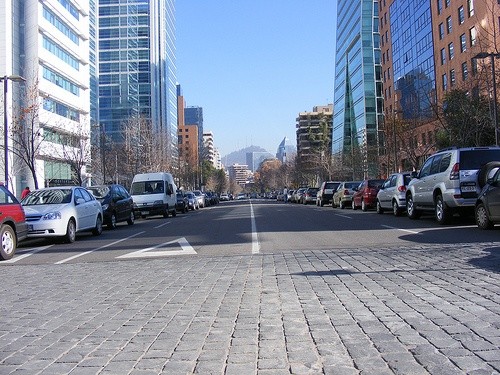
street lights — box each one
[393,108,404,173]
[471,51,500,146]
[91,123,105,183]
[0,75,26,204]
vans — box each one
[130,172,177,218]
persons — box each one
[22,186,30,199]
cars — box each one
[475,167,500,229]
[250,187,321,205]
[185,191,199,211]
[85,184,135,230]
[0,182,28,261]
[201,191,235,207]
[351,179,385,211]
[332,181,363,209]
[193,190,205,208]
[176,189,189,214]
[238,193,245,200]
[19,186,104,244]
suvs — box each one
[405,144,500,225]
[376,170,421,217]
[315,181,342,207]
